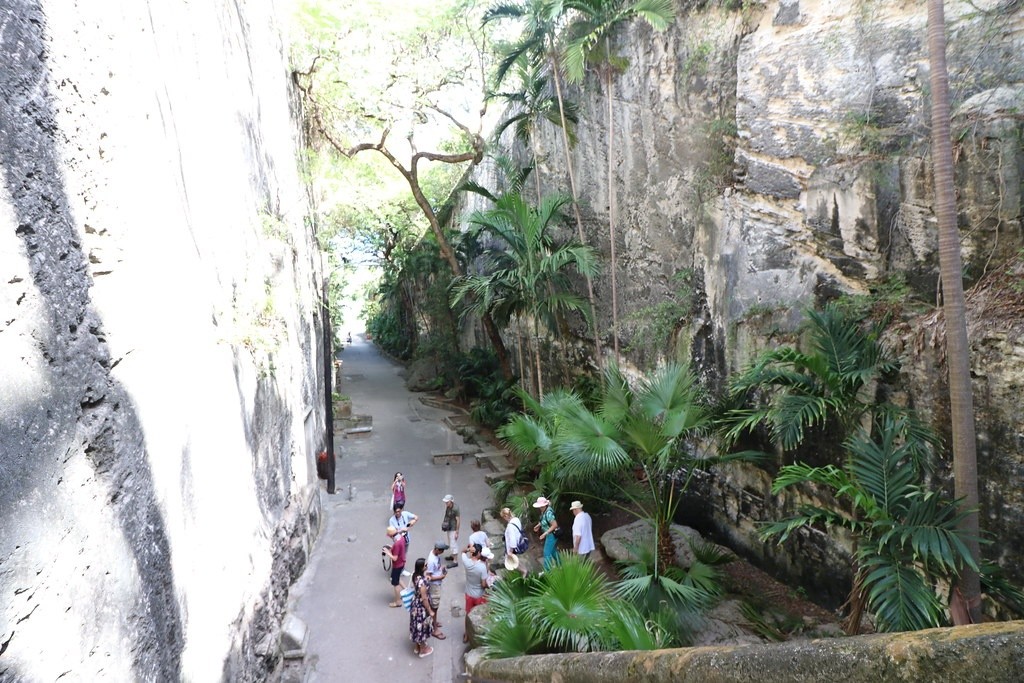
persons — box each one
[500,507,528,577]
[570,501,596,565]
[386,473,491,659]
[532,496,561,578]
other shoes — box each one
[445,556,454,560]
[389,601,402,607]
[463,633,468,644]
[447,562,458,568]
[414,647,434,657]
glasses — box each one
[396,511,400,513]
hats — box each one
[435,542,449,549]
[570,501,583,510]
[442,494,455,502]
[533,497,549,508]
[481,547,494,559]
[504,554,519,571]
[387,526,397,536]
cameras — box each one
[381,546,390,556]
[403,534,409,543]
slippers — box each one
[431,632,446,639]
[436,622,443,627]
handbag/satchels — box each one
[538,526,544,541]
[390,494,394,511]
[517,529,529,554]
[546,510,565,538]
[442,519,451,531]
[399,572,416,612]
